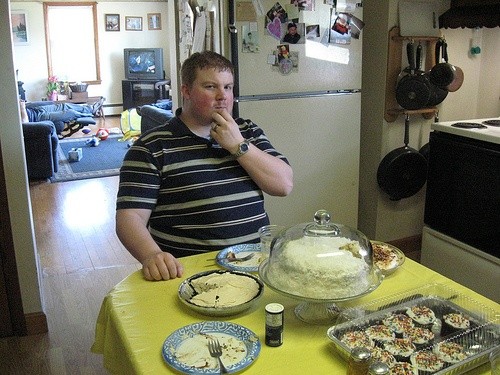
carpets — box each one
[48,131,132,183]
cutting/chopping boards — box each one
[398,1,440,37]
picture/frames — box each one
[106,14,120,31]
[10,10,29,45]
[147,13,162,30]
[125,16,142,31]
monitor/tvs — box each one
[124,48,163,80]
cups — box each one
[258,225,286,262]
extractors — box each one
[438,0,500,30]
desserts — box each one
[338,306,471,375]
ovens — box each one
[423,130,500,269]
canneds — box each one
[264,303,284,347]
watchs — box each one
[234,139,250,157]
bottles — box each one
[265,303,284,347]
[346,348,390,375]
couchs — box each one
[22,119,59,181]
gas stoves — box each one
[432,114,500,145]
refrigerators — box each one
[228,0,363,233]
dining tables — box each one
[89,238,500,374]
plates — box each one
[178,269,265,317]
[369,239,405,276]
[162,321,261,375]
[217,243,261,271]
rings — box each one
[214,124,220,131]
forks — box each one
[206,253,255,262]
[208,339,228,374]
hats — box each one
[287,23,297,29]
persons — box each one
[129,55,155,73]
[246,0,350,58]
[20,99,93,123]
[115,50,293,282]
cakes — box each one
[265,236,371,299]
[188,271,263,308]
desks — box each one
[57,96,106,120]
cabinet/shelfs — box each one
[121,78,166,112]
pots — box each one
[377,113,428,201]
[395,38,464,110]
